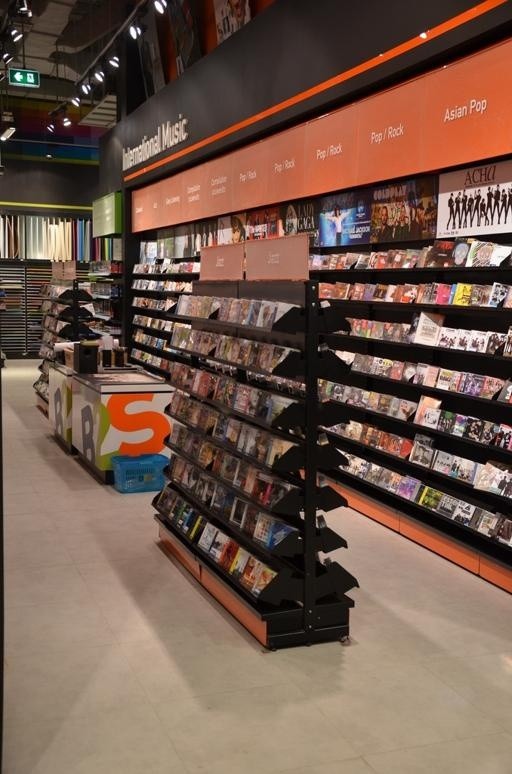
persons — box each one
[446,184,511,230]
[325,207,352,245]
[230,216,245,243]
[285,205,297,236]
[377,201,424,242]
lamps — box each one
[70,53,120,108]
[0,1,32,83]
[45,109,72,134]
[153,1,168,15]
[129,16,143,40]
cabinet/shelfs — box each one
[150,234,359,652]
[35,261,177,485]
[121,234,511,590]
